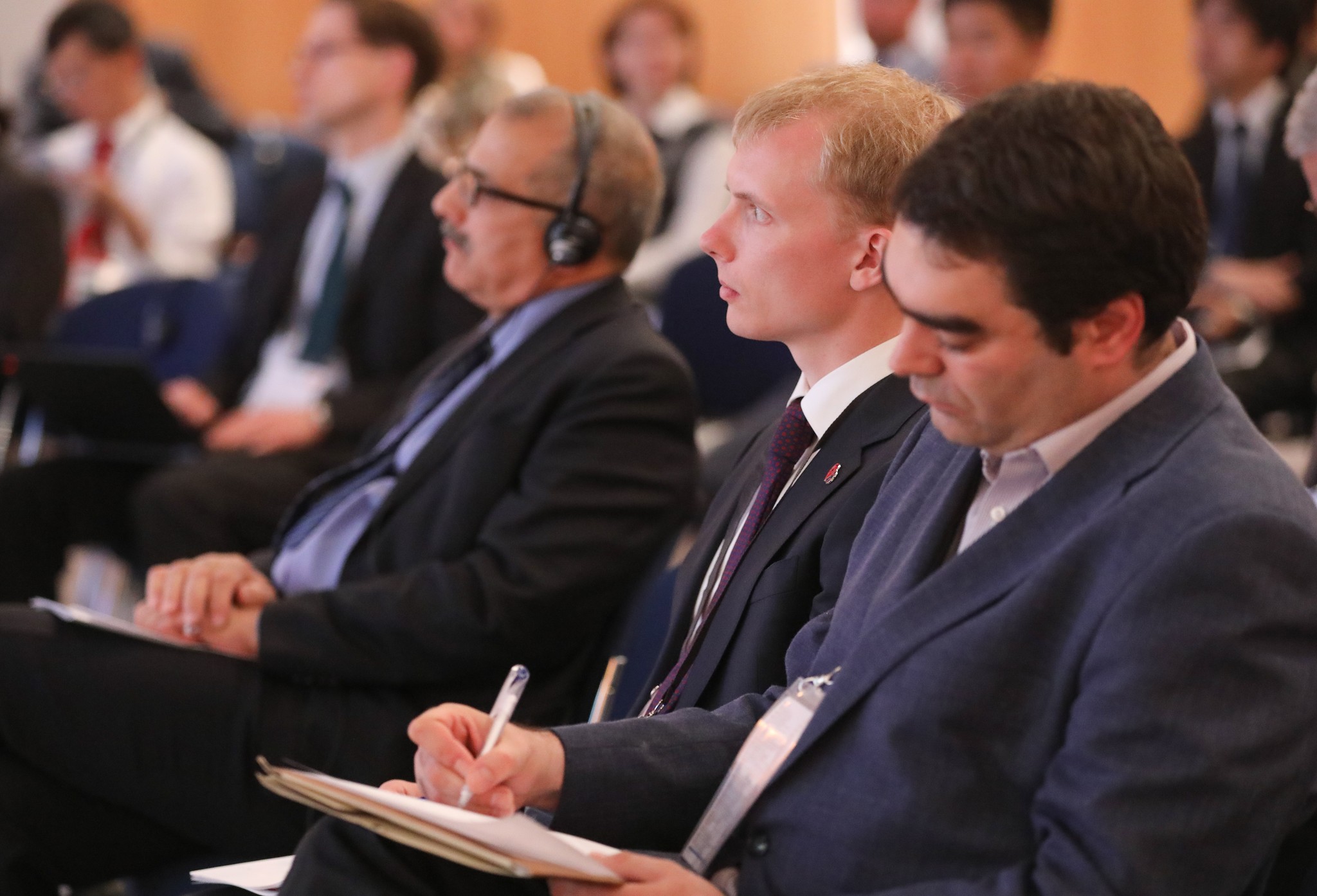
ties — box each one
[301,182,353,359]
[66,131,114,267]
[642,397,818,718]
[257,327,496,574]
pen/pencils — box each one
[590,657,628,722]
[459,665,529,811]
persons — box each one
[0,0,1317,501]
[546,67,1017,878]
[278,80,1317,896]
[0,87,698,896]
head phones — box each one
[544,89,601,263]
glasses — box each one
[458,165,595,224]
